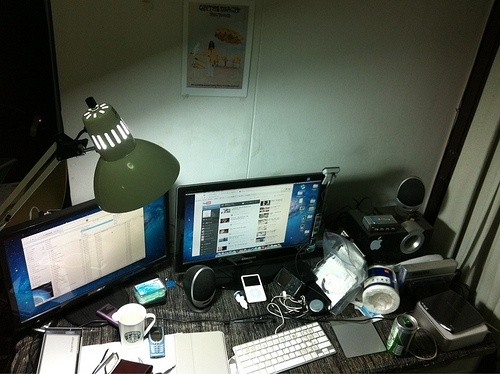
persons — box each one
[208,40,217,80]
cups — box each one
[112,303,156,350]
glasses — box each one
[91,349,119,374]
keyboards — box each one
[232,321,337,374]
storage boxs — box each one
[399,258,458,291]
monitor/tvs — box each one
[0,189,173,331]
[176,172,326,289]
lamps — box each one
[0,96,179,232]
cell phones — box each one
[149,326,165,359]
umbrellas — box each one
[214,27,242,58]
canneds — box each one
[386,314,418,357]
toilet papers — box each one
[361,265,400,316]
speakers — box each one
[184,264,217,313]
[395,176,426,217]
[345,205,434,266]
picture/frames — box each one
[181,0,255,97]
